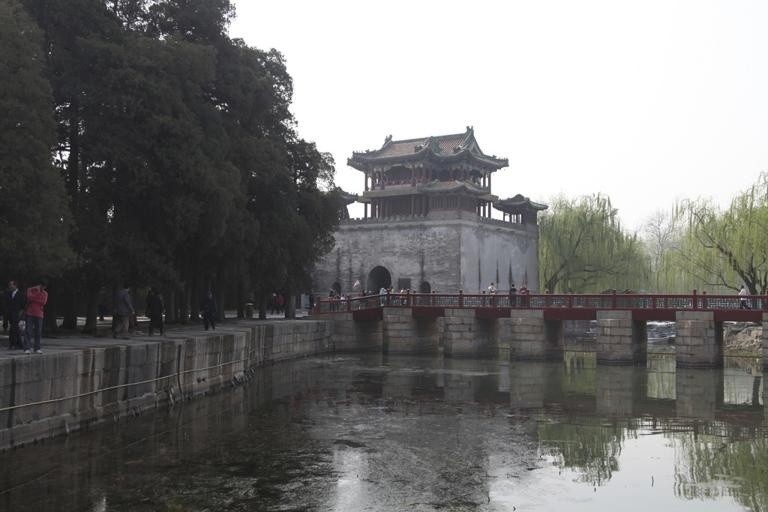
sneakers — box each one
[25,348,31,355]
[35,349,43,354]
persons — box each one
[328,287,346,312]
[269,291,285,316]
[360,286,409,306]
[201,289,218,331]
[487,282,496,294]
[245,295,257,319]
[148,291,165,335]
[110,284,135,340]
[0,276,49,354]
[738,284,751,309]
[507,283,530,305]
